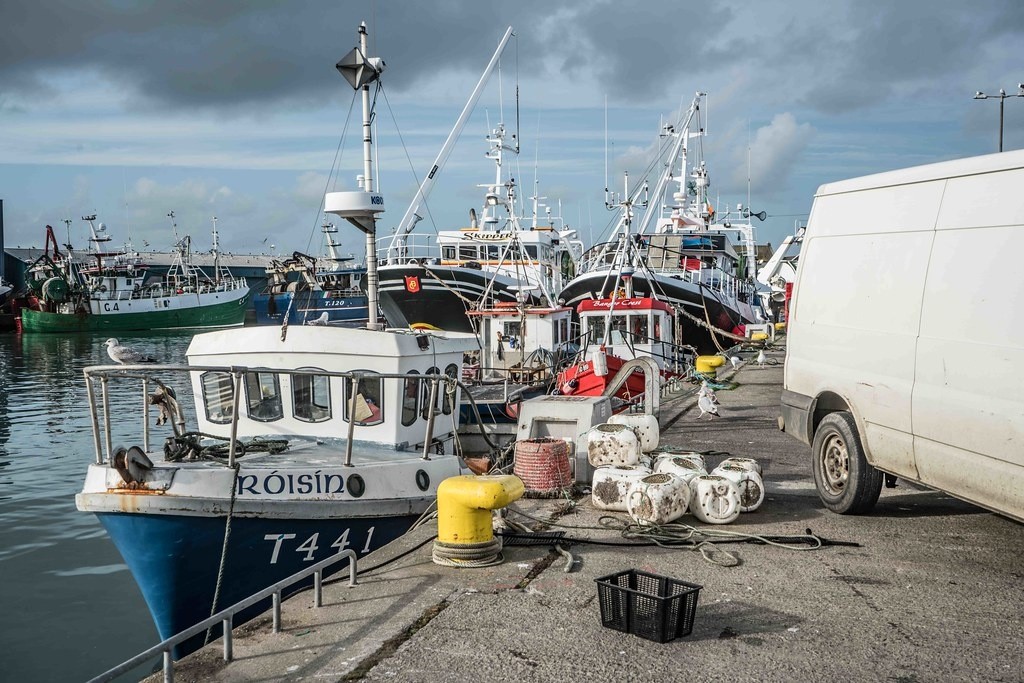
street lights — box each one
[973,83,1024,152]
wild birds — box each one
[757,350,768,369]
[731,356,744,371]
[695,380,722,421]
[102,336,143,374]
[304,311,329,328]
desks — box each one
[508,363,546,382]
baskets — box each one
[594,568,703,644]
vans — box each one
[777,150,1024,527]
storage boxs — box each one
[558,356,685,416]
[462,363,479,387]
[596,568,704,643]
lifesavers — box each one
[609,290,626,299]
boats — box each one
[1,19,804,660]
